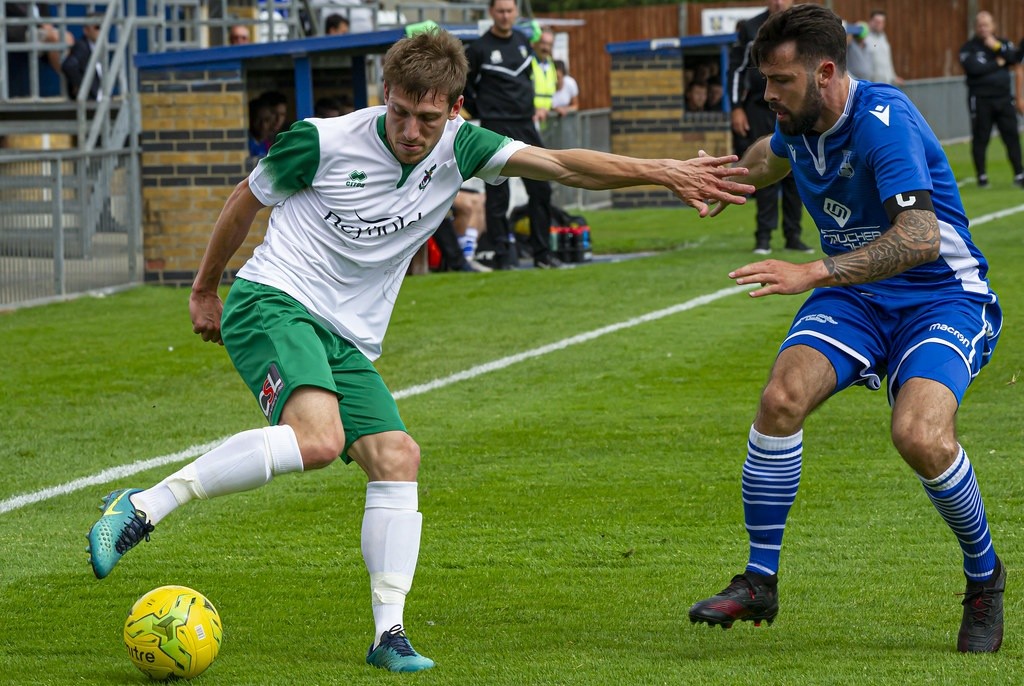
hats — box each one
[838,20,869,40]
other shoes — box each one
[784,241,814,254]
[441,256,479,273]
[460,259,492,272]
[754,236,772,254]
[534,255,561,268]
[1013,172,1024,189]
[493,261,519,271]
[978,175,988,188]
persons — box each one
[461,0,566,271]
[326,14,349,35]
[685,58,722,113]
[697,1,1012,653]
[735,11,905,90]
[959,10,1024,187]
[229,25,250,45]
[249,90,495,274]
[0,0,129,233]
[531,26,579,120]
[725,0,818,255]
[88,29,758,672]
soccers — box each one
[123,584,223,681]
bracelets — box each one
[993,40,1002,51]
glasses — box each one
[231,36,246,41]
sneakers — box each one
[689,571,780,632]
[86,488,154,580]
[957,552,1007,655]
[365,631,434,673]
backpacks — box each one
[508,203,594,264]
